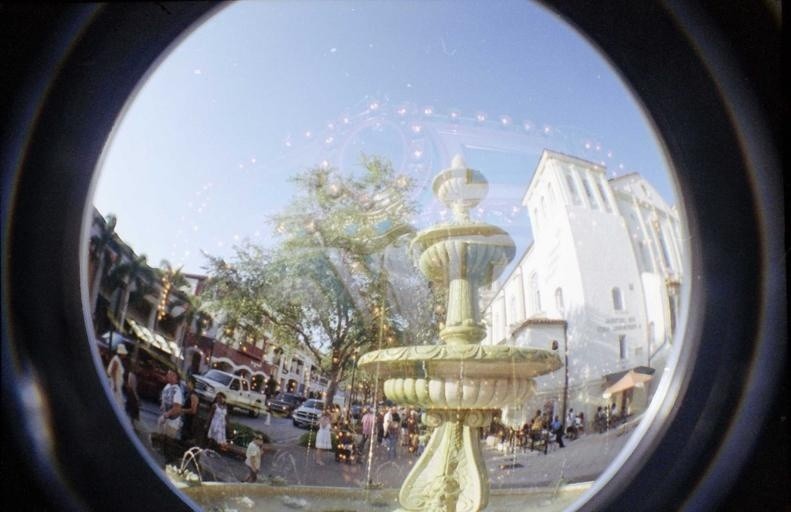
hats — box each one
[117,344,128,354]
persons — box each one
[107,343,128,410]
[155,368,183,460]
[176,375,201,461]
[521,400,622,448]
[125,361,144,432]
[314,414,332,467]
[357,404,422,461]
[203,392,230,458]
[242,433,266,484]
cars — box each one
[94,330,363,431]
